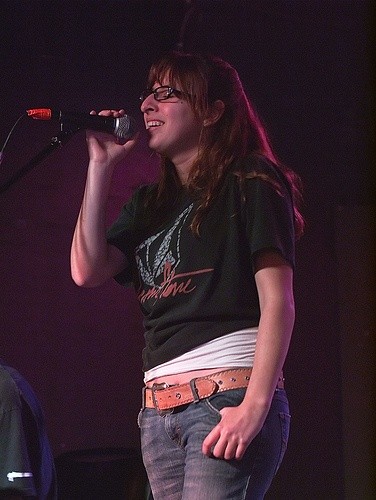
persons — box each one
[0,366,35,500]
[70,53,305,500]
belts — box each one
[145,366,284,411]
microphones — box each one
[24,107,136,140]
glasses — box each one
[139,86,197,101]
